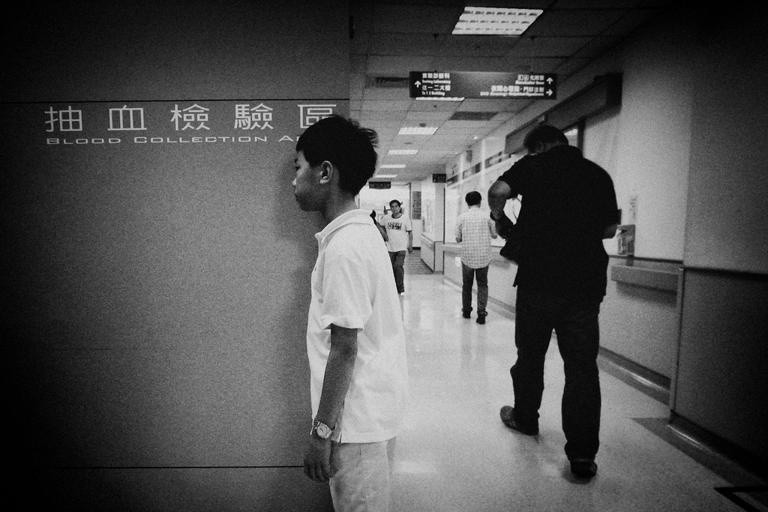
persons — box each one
[487,124,617,481]
[455,191,498,323]
[377,199,413,297]
[292,116,408,512]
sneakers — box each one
[476,316,486,325]
[462,311,471,318]
[500,405,539,436]
[570,461,597,484]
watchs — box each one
[312,419,337,440]
